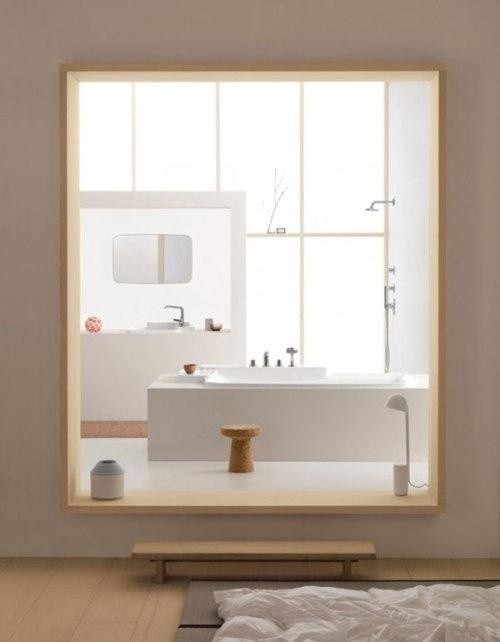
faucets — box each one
[263,351,270,366]
[286,346,299,366]
[165,304,184,326]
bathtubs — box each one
[147,366,429,464]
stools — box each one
[218,422,262,473]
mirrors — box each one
[113,234,192,283]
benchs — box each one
[130,540,375,585]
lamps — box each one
[384,394,411,495]
